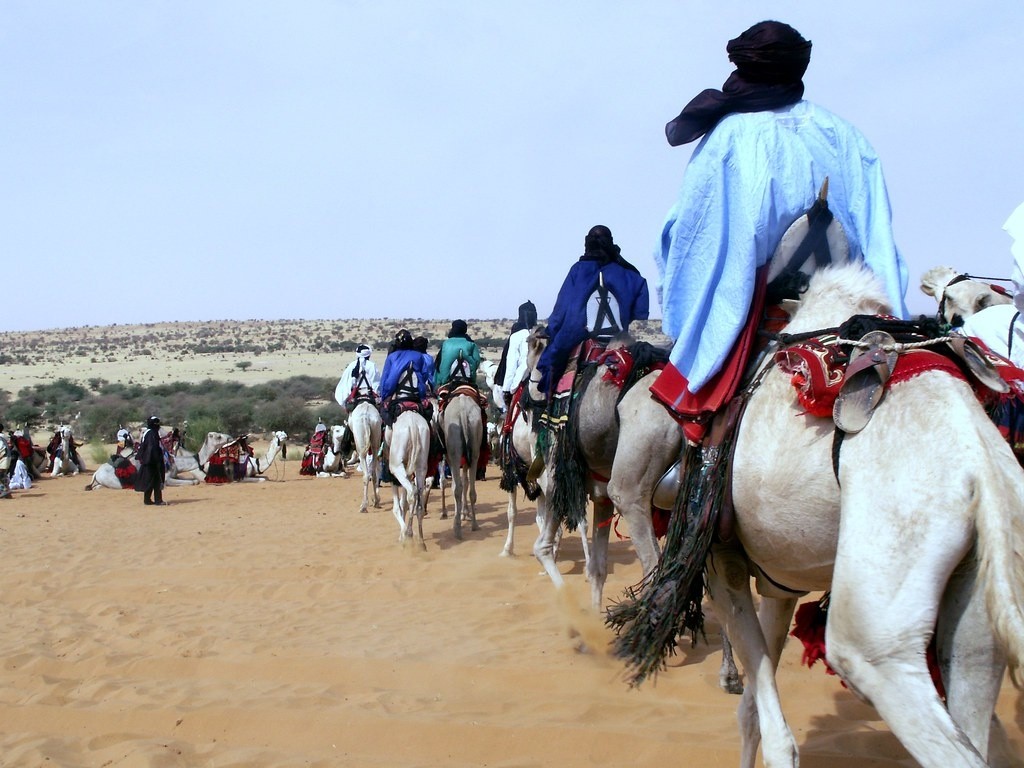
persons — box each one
[493,300,538,399]
[433,319,481,404]
[944,201,1024,393]
[0,423,12,499]
[379,329,428,425]
[335,344,382,409]
[413,336,435,390]
[135,416,169,505]
[649,21,911,444]
[536,225,649,393]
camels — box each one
[85,420,288,491]
[9,425,85,477]
[301,267,1023,768]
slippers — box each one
[833,331,908,436]
[940,327,1011,395]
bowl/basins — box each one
[650,458,685,511]
[526,454,545,482]
